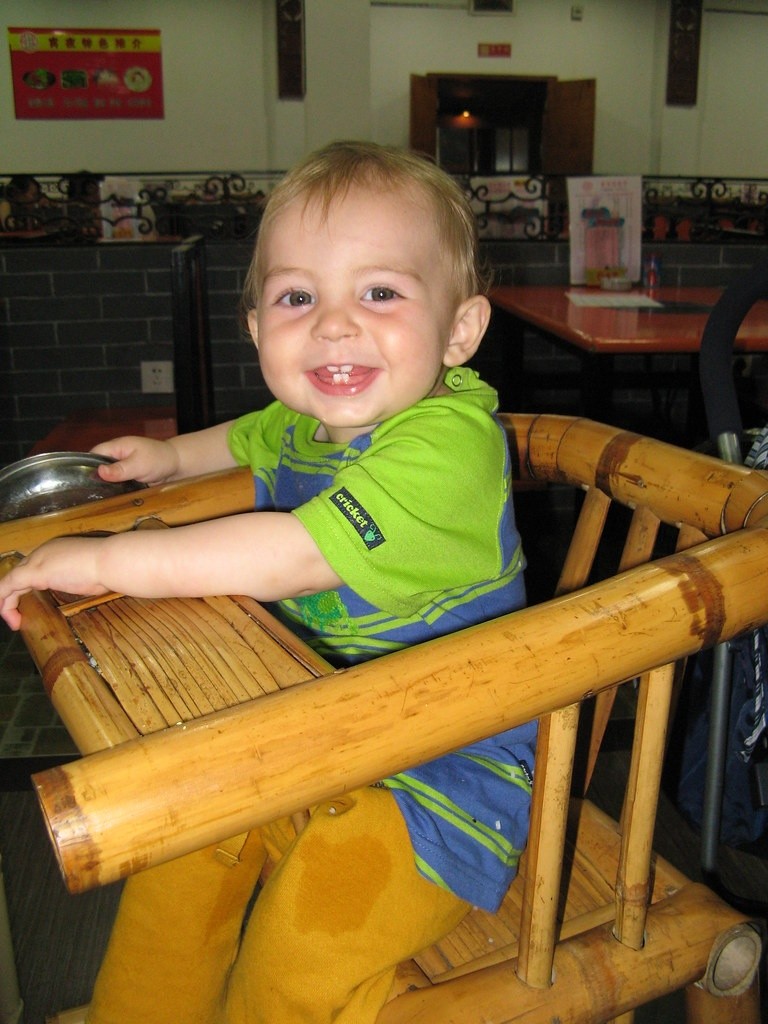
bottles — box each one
[643,253,661,289]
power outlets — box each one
[142,361,173,394]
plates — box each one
[60,69,89,91]
[123,66,152,93]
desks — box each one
[476,285,768,432]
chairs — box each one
[0,413,767,1024]
[25,234,217,458]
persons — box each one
[0,176,49,231]
[67,171,156,232]
[0,140,537,1024]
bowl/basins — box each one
[1,451,149,523]
[22,70,56,90]
